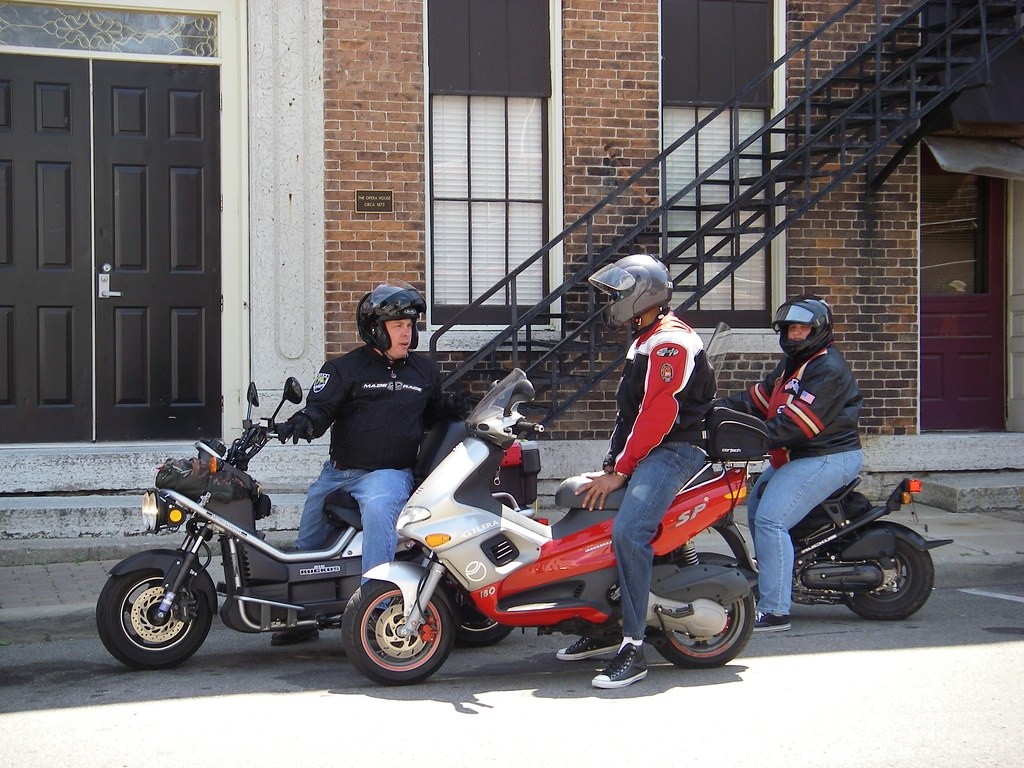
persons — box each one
[710,296,863,630]
[269,279,449,647]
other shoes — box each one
[270,629,320,645]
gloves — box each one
[274,414,314,446]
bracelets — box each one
[612,471,629,479]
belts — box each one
[329,456,346,471]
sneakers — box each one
[592,642,648,689]
[753,613,792,632]
[556,635,623,661]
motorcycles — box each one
[96,377,541,670]
[706,322,954,621]
[341,367,770,687]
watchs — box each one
[556,255,717,689]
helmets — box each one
[603,254,673,333]
[777,295,833,360]
[356,278,427,351]
